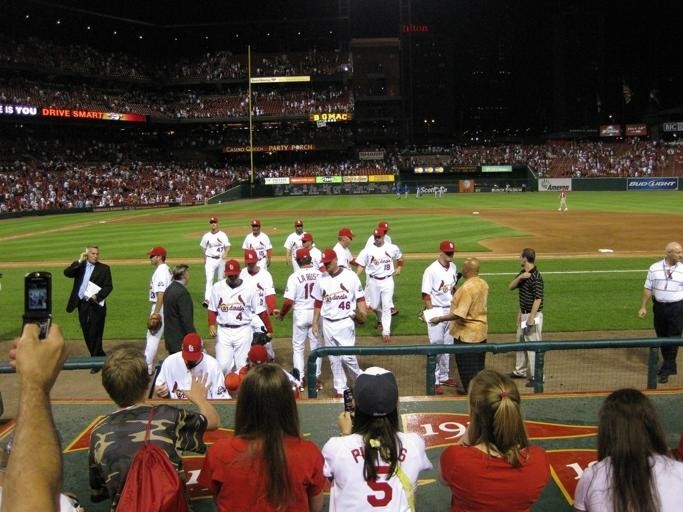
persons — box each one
[142,246,173,375]
[571,388,682,511]
[87,344,224,512]
[205,364,325,512]
[154,332,231,399]
[0,324,69,511]
[199,215,403,398]
[161,261,195,353]
[429,256,488,397]
[636,240,683,383]
[319,364,430,512]
[419,240,460,396]
[438,369,550,511]
[62,244,112,375]
[507,248,545,387]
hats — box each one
[146,246,166,257]
[294,220,304,226]
[373,222,391,237]
[225,259,240,277]
[339,228,357,240]
[244,249,258,264]
[439,240,454,253]
[251,219,260,226]
[298,233,313,242]
[319,249,337,263]
[293,247,310,258]
[248,344,268,364]
[209,217,219,223]
[181,333,204,362]
[355,366,398,417]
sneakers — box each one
[436,377,458,395]
[506,367,545,386]
[656,366,678,385]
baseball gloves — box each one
[252,326,270,345]
[349,310,367,324]
[147,314,161,336]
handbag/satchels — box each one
[115,446,189,511]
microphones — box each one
[452,271,462,289]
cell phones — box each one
[20,271,52,341]
[344,390,354,415]
